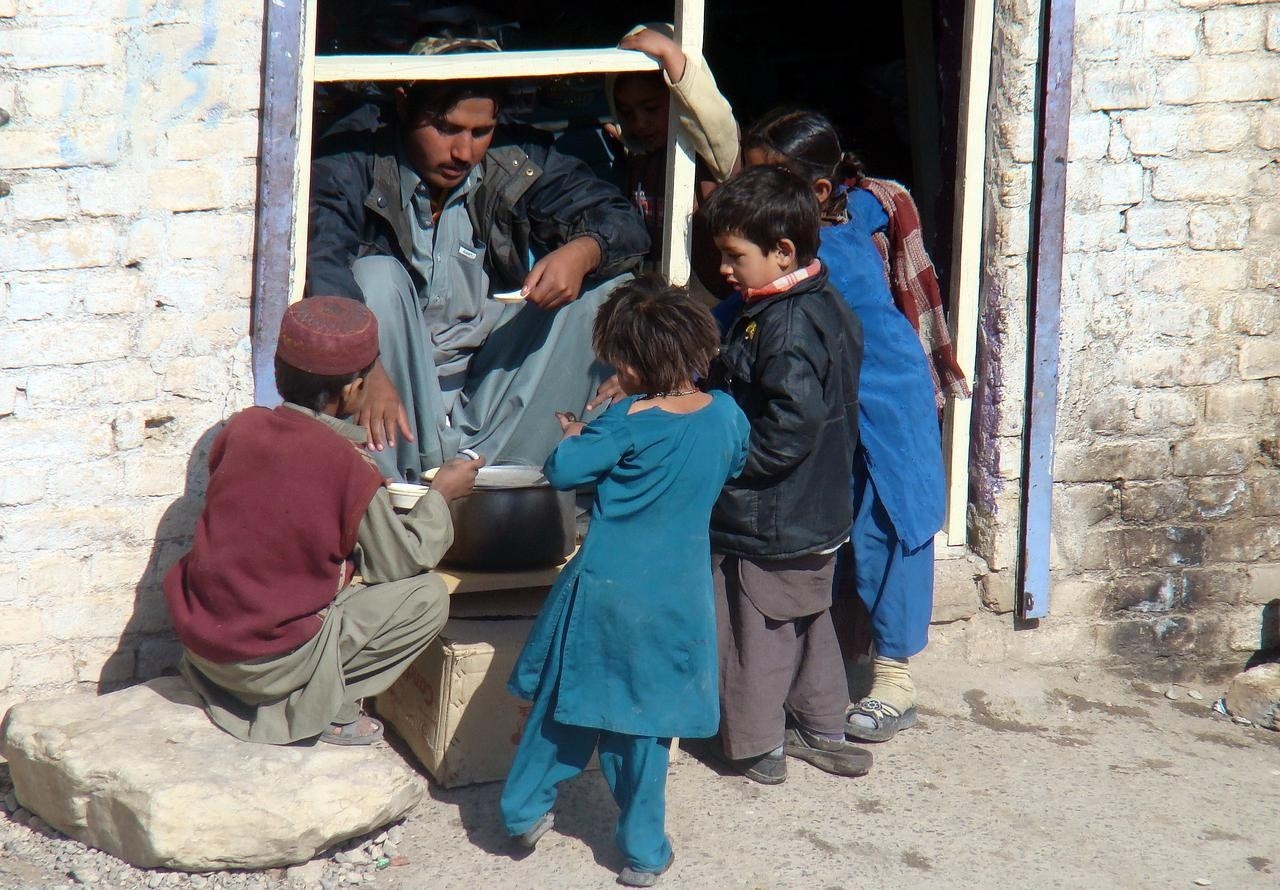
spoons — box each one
[493,289,533,303]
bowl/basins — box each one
[387,483,430,509]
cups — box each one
[393,39,407,50]
[328,35,343,50]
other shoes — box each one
[708,737,787,784]
[620,847,674,886]
[515,811,557,846]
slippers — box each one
[312,716,385,745]
[844,698,920,737]
[781,716,872,775]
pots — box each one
[419,465,576,572]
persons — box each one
[305,37,651,485]
[161,296,486,745]
[554,22,737,184]
[742,107,946,743]
[500,275,751,886]
[692,164,872,786]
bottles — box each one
[321,16,353,50]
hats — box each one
[276,297,380,376]
[401,38,500,89]
